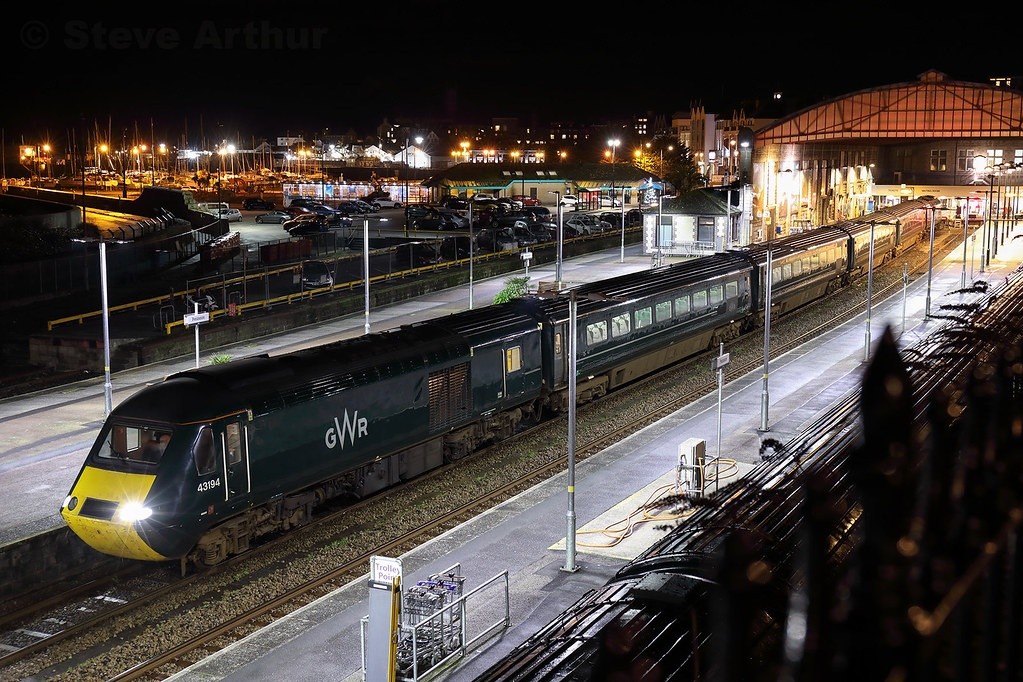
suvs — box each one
[242,198,276,211]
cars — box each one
[395,194,622,270]
[254,197,404,233]
[181,186,217,193]
[215,208,243,223]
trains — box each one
[57,196,941,575]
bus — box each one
[197,203,229,214]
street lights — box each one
[986,160,1023,258]
[405,135,424,237]
[742,244,810,431]
[915,206,952,320]
[636,143,651,169]
[539,289,610,571]
[343,216,392,335]
[658,196,676,267]
[68,236,134,420]
[548,190,559,282]
[956,197,982,287]
[450,204,498,310]
[854,220,903,361]
[717,189,740,251]
[731,140,738,169]
[608,139,621,161]
[661,145,673,178]
[614,186,633,263]
[975,190,998,272]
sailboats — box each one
[12,118,328,187]
